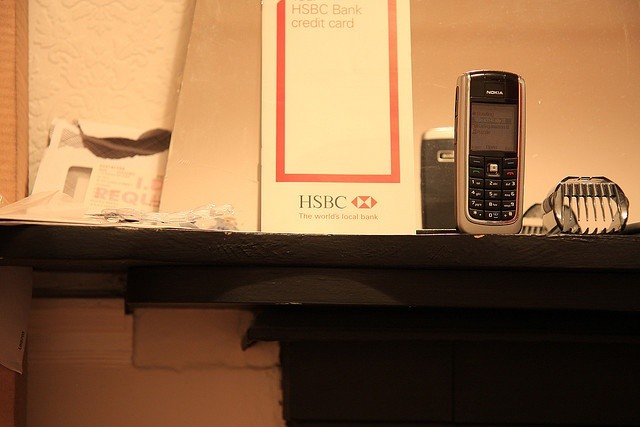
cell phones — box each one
[454,69,526,236]
[421,126,454,230]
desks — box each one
[0,216,638,424]
